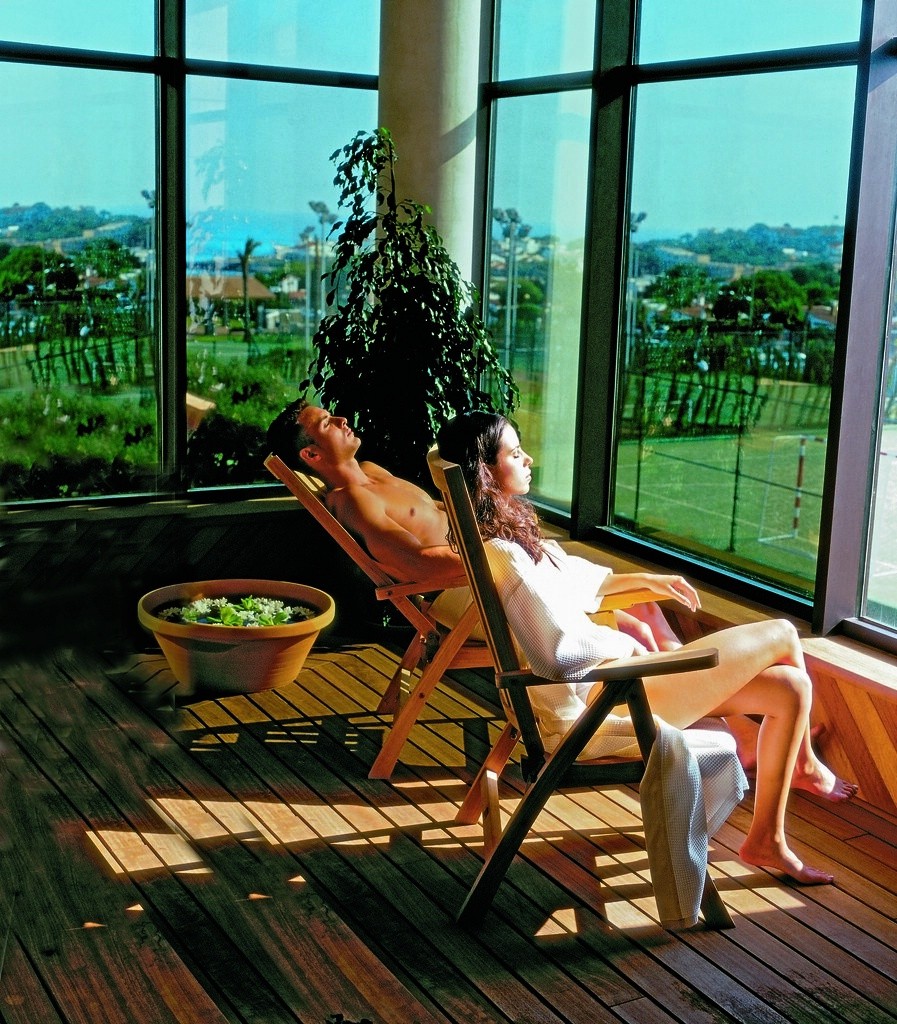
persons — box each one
[266,397,825,769]
[437,411,858,884]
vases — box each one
[137,579,337,693]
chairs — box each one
[264,452,496,781]
[426,442,736,930]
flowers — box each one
[157,594,320,628]
